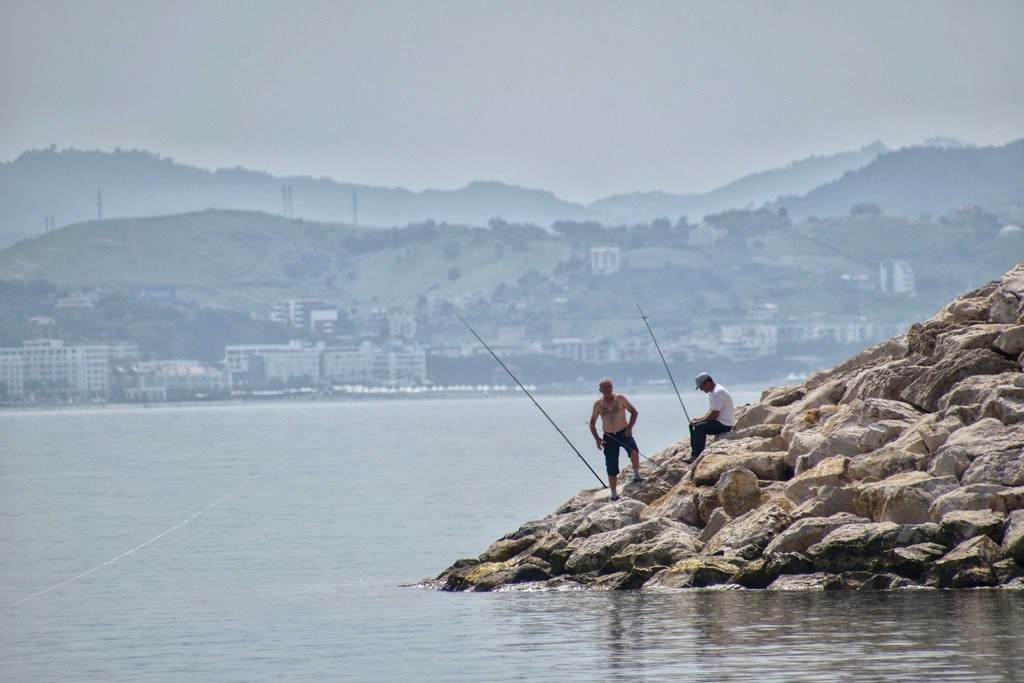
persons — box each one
[590,379,646,501]
[682,372,734,464]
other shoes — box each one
[682,455,697,463]
[610,496,619,501]
[633,477,645,482]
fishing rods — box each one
[454,311,608,489]
[583,419,681,481]
[635,302,690,421]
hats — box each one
[695,372,709,390]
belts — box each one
[604,428,626,435]
